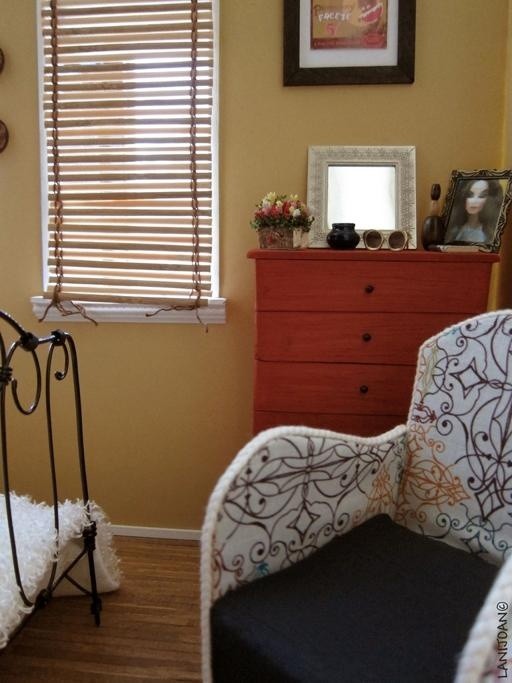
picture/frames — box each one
[437,168,511,253]
[282,0,416,86]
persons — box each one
[444,177,504,246]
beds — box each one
[0,308,121,683]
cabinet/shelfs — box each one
[246,245,501,436]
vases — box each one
[326,223,360,250]
[257,227,308,249]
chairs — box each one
[200,308,512,683]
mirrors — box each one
[307,144,418,249]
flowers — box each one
[250,191,313,245]
